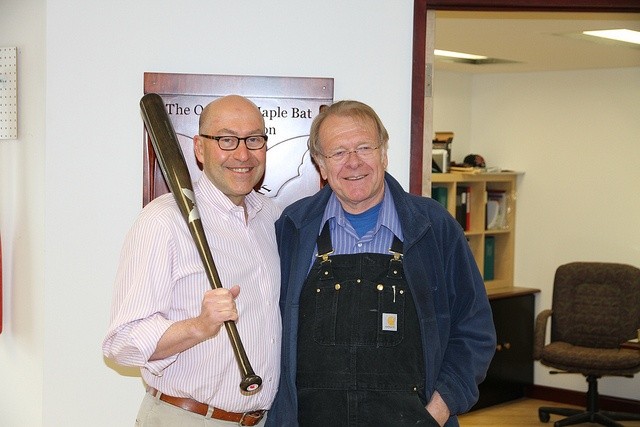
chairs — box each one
[534,261,637,427]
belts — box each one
[145,385,266,426]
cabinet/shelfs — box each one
[424,169,518,297]
[456,284,541,413]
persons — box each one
[264,101,498,426]
[101,94,282,426]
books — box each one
[456,185,471,230]
[431,186,447,208]
[451,163,486,174]
[485,189,507,228]
[485,236,494,280]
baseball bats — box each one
[140,94,263,392]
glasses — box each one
[317,142,381,162]
[199,133,268,151]
